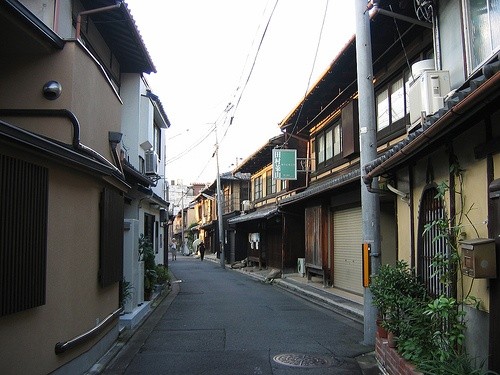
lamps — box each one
[43,80,62,100]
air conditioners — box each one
[144,151,158,175]
[409,71,451,125]
[243,200,249,211]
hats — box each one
[172,238,176,241]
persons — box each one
[170,238,178,262]
[200,242,206,262]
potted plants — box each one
[155,265,172,290]
[370,259,429,347]
